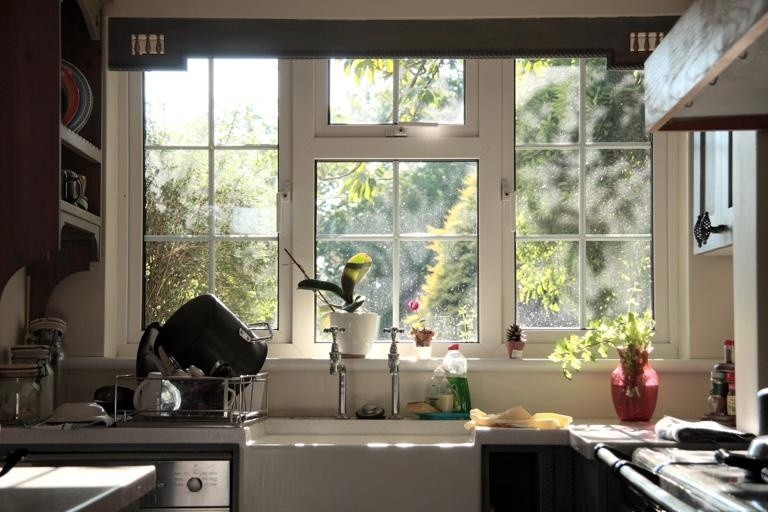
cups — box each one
[134,370,163,415]
[62,166,89,209]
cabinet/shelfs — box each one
[475,441,664,510]
[1,1,105,326]
[689,128,736,258]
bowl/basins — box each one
[48,400,110,423]
[94,385,134,414]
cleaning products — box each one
[425,358,454,413]
[443,344,471,413]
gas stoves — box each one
[597,444,767,511]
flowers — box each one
[402,298,432,331]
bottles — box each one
[424,344,474,414]
[1,339,56,424]
[704,336,736,421]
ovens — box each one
[11,445,237,512]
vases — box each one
[409,329,436,347]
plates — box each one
[60,58,95,134]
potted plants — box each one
[504,322,526,359]
[297,250,382,361]
[547,310,663,423]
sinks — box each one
[238,418,483,512]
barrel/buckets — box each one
[154,292,273,403]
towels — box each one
[655,415,755,450]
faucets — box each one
[382,327,405,418]
[323,325,350,419]
